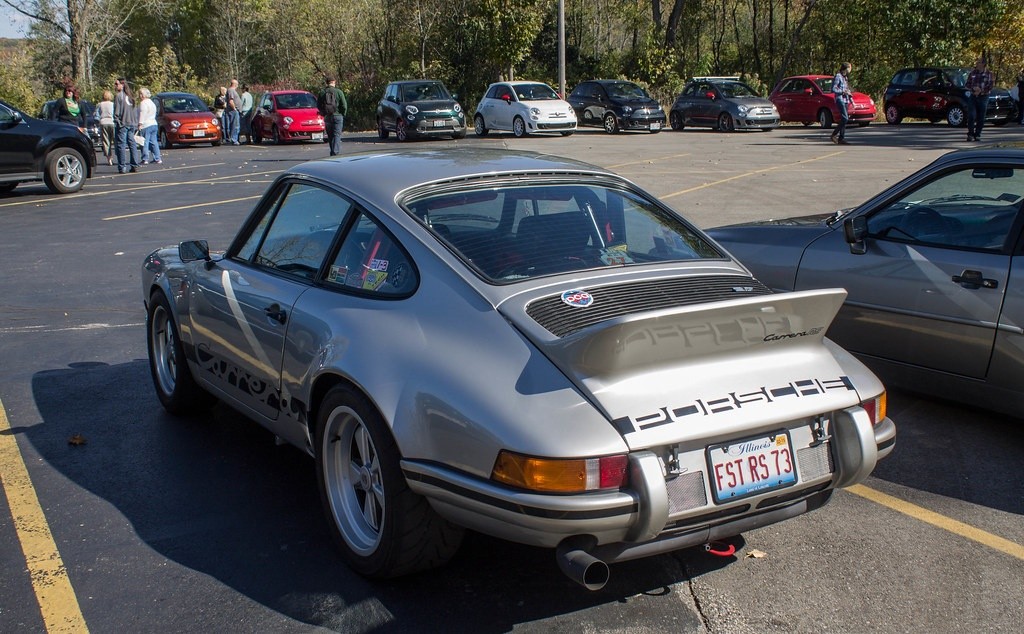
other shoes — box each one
[1017,121,1024,125]
[830,134,839,144]
[108,162,114,166]
[138,160,148,165]
[975,137,981,142]
[129,165,140,173]
[150,158,162,164]
[118,167,126,173]
[966,135,975,142]
[221,136,264,147]
[837,138,849,145]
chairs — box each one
[511,211,592,263]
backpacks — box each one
[320,86,338,116]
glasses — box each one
[64,88,73,92]
[114,82,121,86]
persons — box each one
[966,57,993,139]
[317,77,347,157]
[1017,71,1024,124]
[96,91,115,166]
[240,83,254,145]
[113,78,140,174]
[137,88,163,165]
[831,63,851,144]
[53,83,88,133]
[227,79,242,146]
[214,87,231,144]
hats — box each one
[326,76,336,83]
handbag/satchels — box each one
[1010,82,1020,102]
[215,108,224,118]
[134,130,145,148]
[93,103,101,123]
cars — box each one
[251,90,330,145]
[767,74,876,129]
[474,81,578,139]
[376,80,467,142]
[883,66,1016,129]
[646,147,1024,417]
[141,145,898,592]
[669,76,780,134]
[38,101,103,148]
[0,100,97,195]
[149,91,222,149]
[565,79,667,135]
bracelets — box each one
[138,124,141,126]
